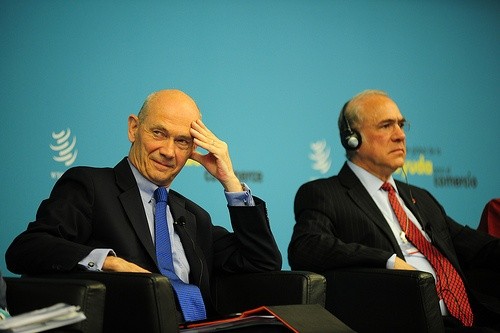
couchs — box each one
[3,270,444,333]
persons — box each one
[5,88,283,325]
[286,89,500,333]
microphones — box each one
[426,222,433,231]
[178,216,186,226]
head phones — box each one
[341,101,362,150]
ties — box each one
[381,182,473,326]
[153,187,206,322]
